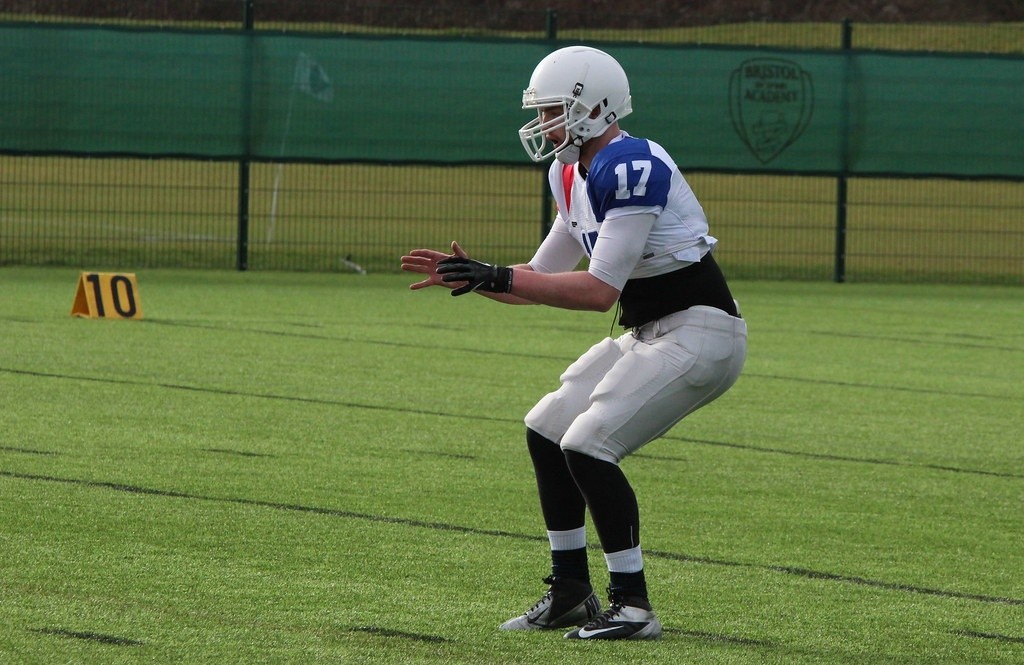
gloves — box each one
[436,257,513,297]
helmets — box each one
[521,46,633,136]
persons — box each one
[400,46,747,640]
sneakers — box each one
[562,584,663,643]
[498,576,603,632]
